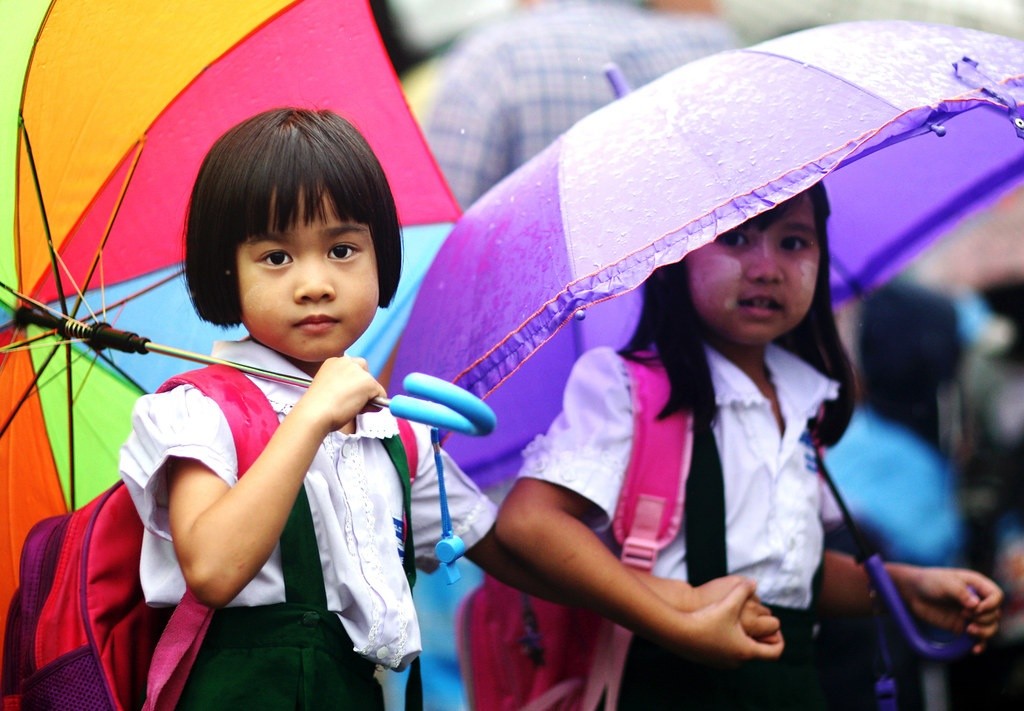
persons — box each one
[423,0,746,209]
[495,181,1024,711]
[120,109,779,711]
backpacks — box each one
[457,347,690,711]
[1,363,418,711]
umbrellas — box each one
[0,1,466,711]
[390,16,1024,667]
